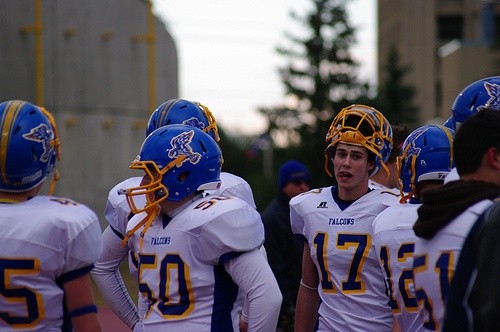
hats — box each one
[279,161,314,190]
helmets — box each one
[325,104,393,178]
[445,76,499,131]
[394,123,456,192]
[138,124,224,201]
[0,99,60,192]
[145,98,220,144]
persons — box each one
[88,77,500,332]
[0,100,103,332]
